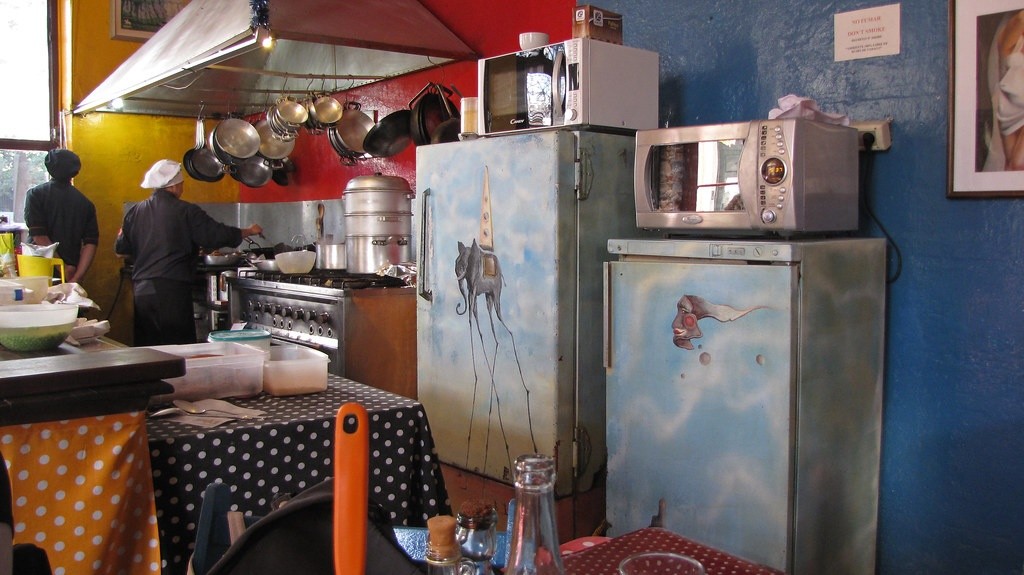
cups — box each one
[618,552,705,575]
[460,97,478,133]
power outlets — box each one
[849,120,893,152]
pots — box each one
[343,173,415,276]
[311,241,347,269]
[327,101,376,166]
[255,91,343,186]
[183,114,273,187]
[363,82,461,157]
[204,247,280,272]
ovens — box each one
[238,324,345,377]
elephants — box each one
[453,239,538,474]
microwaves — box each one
[634,118,859,237]
[478,38,660,136]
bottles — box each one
[507,454,565,575]
[454,497,498,575]
[426,516,476,575]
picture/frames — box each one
[945,0,1024,202]
[109,0,192,44]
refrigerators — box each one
[602,239,888,575]
[415,129,636,499]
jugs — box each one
[17,254,65,287]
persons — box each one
[114,159,263,347]
[24,149,100,284]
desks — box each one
[142,373,453,574]
[540,528,786,575]
[0,409,161,575]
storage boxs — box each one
[571,4,623,46]
[263,343,331,397]
[141,341,271,406]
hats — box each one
[45,149,81,181]
[141,159,184,188]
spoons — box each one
[173,400,266,418]
[150,408,253,420]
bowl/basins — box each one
[519,32,549,51]
[275,251,316,274]
[0,304,79,351]
[7,276,49,303]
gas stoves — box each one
[227,270,407,339]
